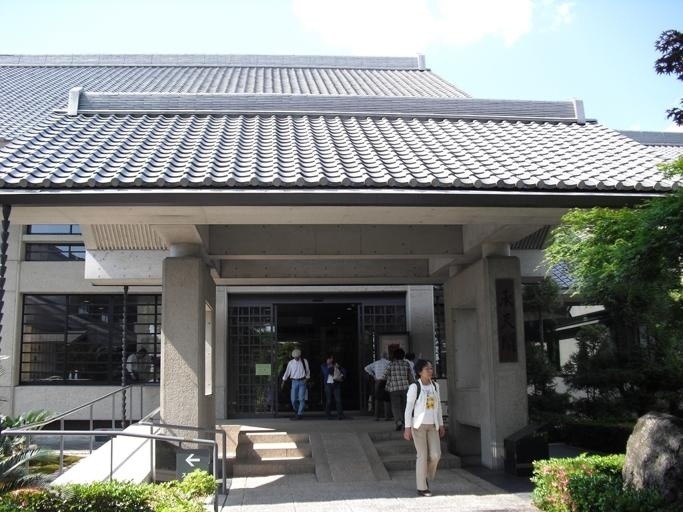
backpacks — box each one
[399,380,436,419]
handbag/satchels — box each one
[333,364,345,382]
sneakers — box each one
[396,421,403,431]
[418,489,432,497]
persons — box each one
[402,359,446,498]
[276,347,415,432]
[124,348,146,382]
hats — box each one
[291,349,301,358]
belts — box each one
[292,377,305,381]
